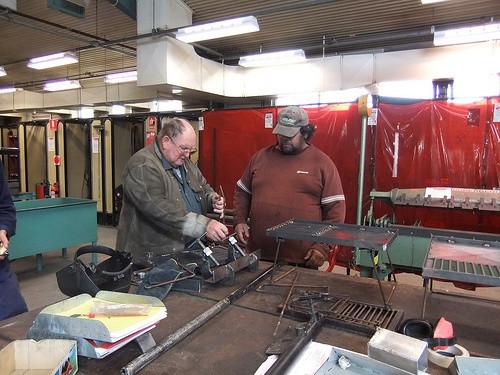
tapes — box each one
[427,341,470,369]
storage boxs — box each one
[0,339,78,375]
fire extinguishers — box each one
[51,182,60,198]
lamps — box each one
[238,49,306,68]
[432,21,499,46]
[27,51,79,70]
[43,79,82,91]
[176,14,260,44]
[104,71,137,84]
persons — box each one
[114,118,229,264]
[232,106,346,271]
[0,160,28,321]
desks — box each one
[0,259,500,375]
